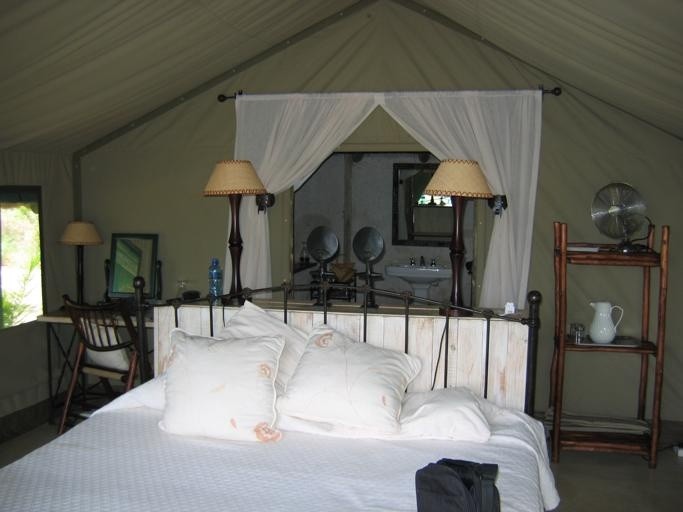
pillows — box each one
[158,328,286,445]
[212,299,492,443]
[277,321,422,442]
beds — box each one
[1,275,561,512]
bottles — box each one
[569,320,587,344]
[208,258,223,304]
[299,241,309,265]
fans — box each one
[589,182,653,254]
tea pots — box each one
[588,299,624,345]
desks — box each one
[37,299,166,426]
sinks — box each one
[385,263,452,284]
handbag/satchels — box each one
[413,456,503,512]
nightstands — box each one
[154,301,530,414]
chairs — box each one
[57,294,141,435]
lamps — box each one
[203,159,268,308]
[60,220,103,313]
[423,158,494,317]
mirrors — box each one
[391,162,454,248]
[106,231,158,298]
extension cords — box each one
[672,445,683,457]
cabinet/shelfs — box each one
[310,269,357,303]
[546,220,670,470]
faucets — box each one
[418,255,426,266]
